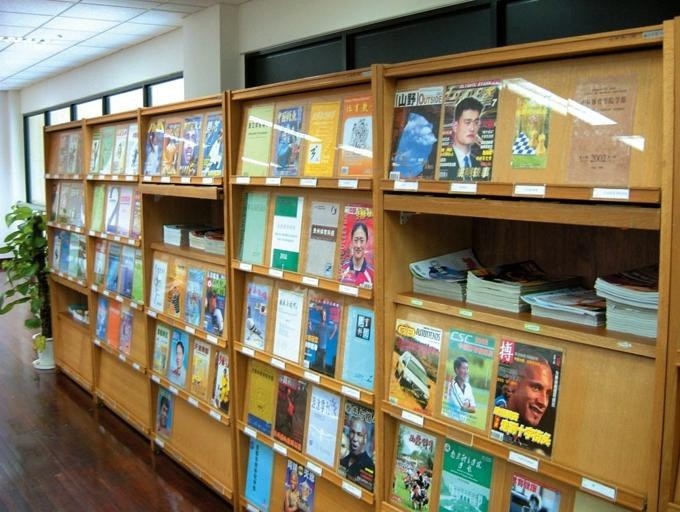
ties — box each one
[464,155,472,181]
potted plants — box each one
[0,201,55,369]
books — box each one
[67,303,88,324]
[163,222,224,256]
[410,248,658,338]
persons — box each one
[171,342,187,385]
[309,309,337,369]
[170,287,179,315]
[338,222,375,286]
[520,493,541,512]
[276,125,299,171]
[284,472,303,512]
[157,396,170,436]
[448,357,475,413]
[440,97,491,181]
[491,348,554,450]
[403,470,430,508]
[340,418,374,479]
[145,125,224,176]
[218,369,229,409]
[208,291,224,336]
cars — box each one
[394,350,431,409]
